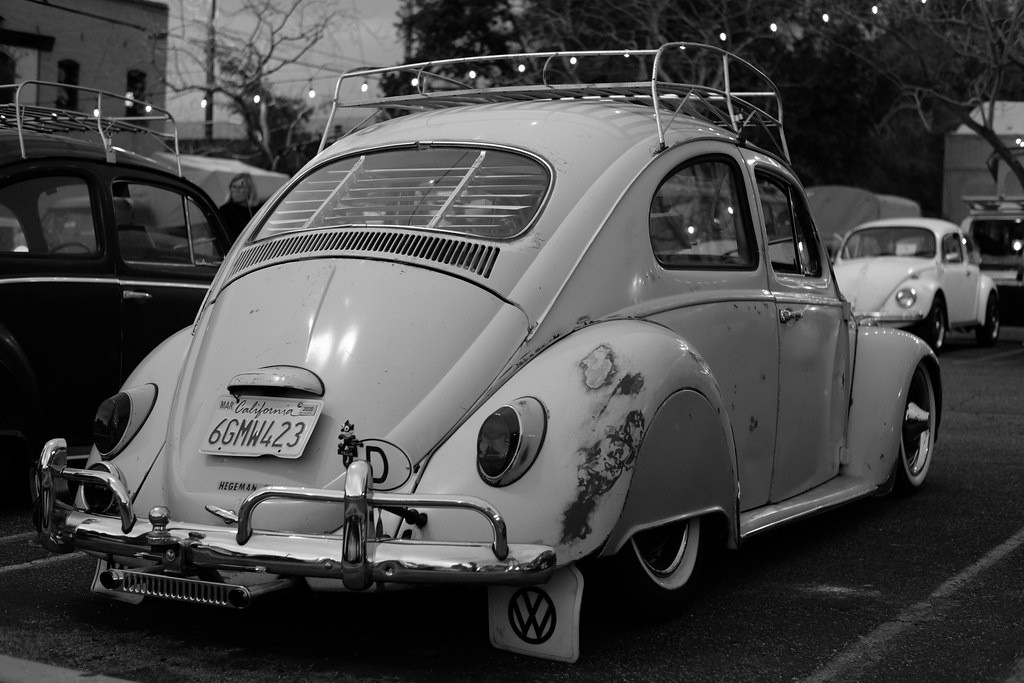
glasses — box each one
[231,185,247,193]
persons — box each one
[212,172,270,246]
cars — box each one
[0,80,234,498]
[958,196,1023,327]
[19,39,950,664]
[832,216,1002,356]
